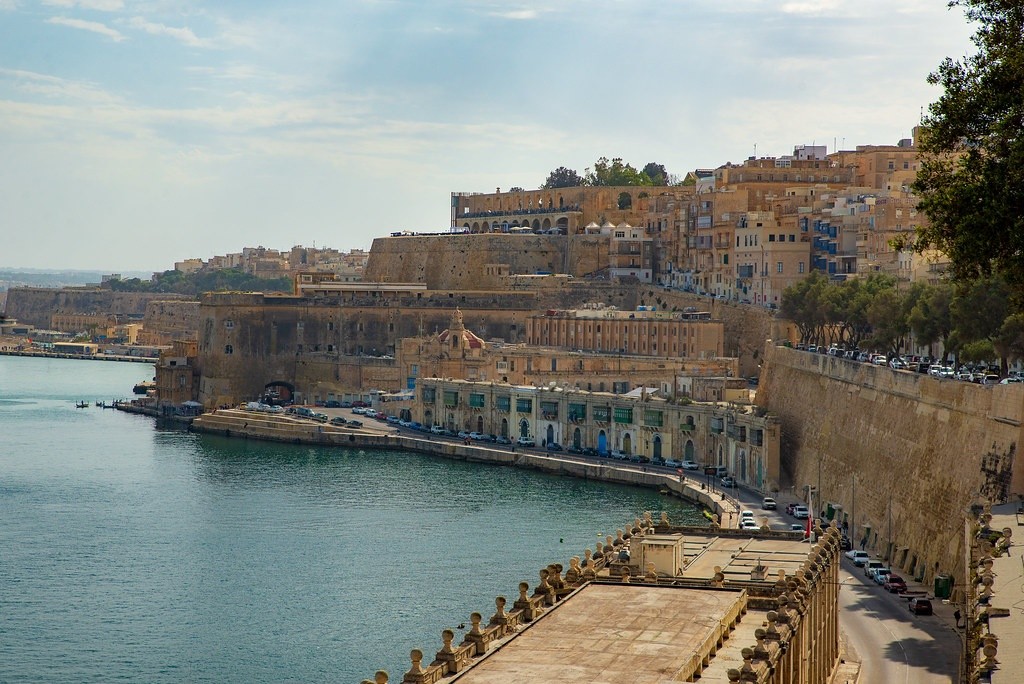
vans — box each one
[715,466,728,478]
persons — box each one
[464,436,471,445]
[954,609,962,628]
[208,407,217,413]
[837,520,841,534]
[860,535,868,551]
[843,520,848,534]
[219,402,235,410]
[397,429,400,436]
[737,487,740,496]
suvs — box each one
[761,497,777,510]
[547,443,563,451]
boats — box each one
[75,400,127,409]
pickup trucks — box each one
[517,437,535,447]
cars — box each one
[785,502,934,616]
[777,338,1024,385]
[748,377,759,386]
[536,271,606,281]
[656,281,777,310]
[720,477,737,488]
[567,446,700,470]
[240,389,513,445]
[739,509,761,531]
